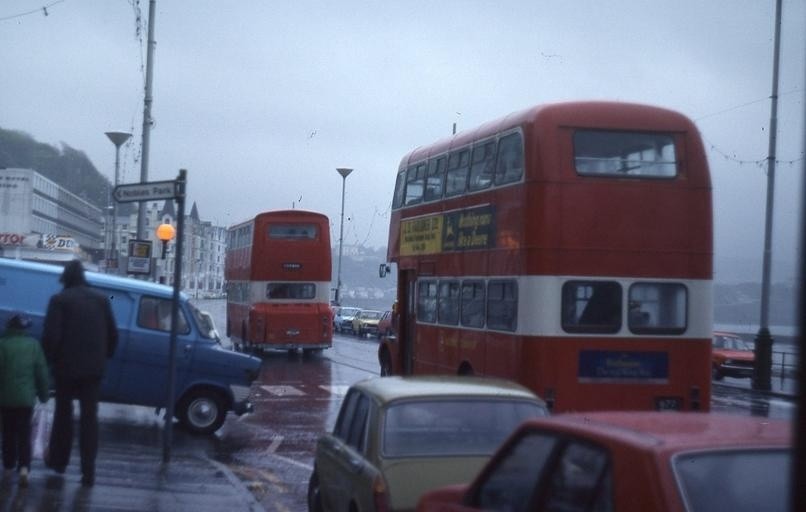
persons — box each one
[42,260,119,489]
[0,312,49,489]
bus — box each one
[379,101,717,415]
[225,209,335,359]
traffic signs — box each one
[112,179,178,203]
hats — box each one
[59,259,85,282]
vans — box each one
[0,254,266,438]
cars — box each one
[197,310,220,342]
[309,377,551,509]
[417,411,792,511]
[712,331,755,381]
[203,287,228,299]
[331,304,395,339]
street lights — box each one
[107,130,133,272]
[158,222,175,287]
[335,167,355,306]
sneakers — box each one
[18,465,29,488]
[4,468,18,484]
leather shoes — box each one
[43,447,66,473]
